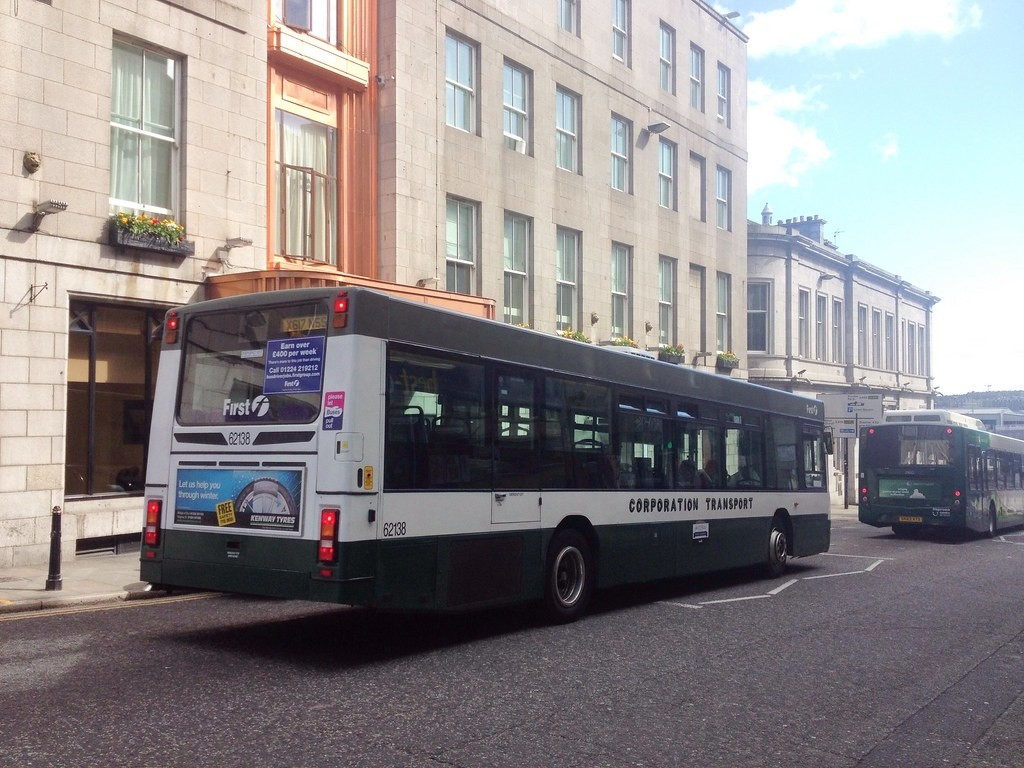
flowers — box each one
[659,344,685,356]
[562,326,591,343]
[612,336,640,349]
[110,212,185,245]
[509,322,529,328]
[717,351,738,363]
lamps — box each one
[934,387,940,390]
[821,273,836,280]
[859,376,866,383]
[723,11,740,19]
[217,237,253,261]
[418,278,440,287]
[29,200,67,231]
[798,370,806,377]
[904,382,910,388]
[648,121,670,134]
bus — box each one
[858,408,1024,538]
[138,286,836,622]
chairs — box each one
[633,457,654,488]
[384,405,608,488]
[677,461,702,488]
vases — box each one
[108,227,196,259]
[658,353,685,365]
[717,359,739,369]
